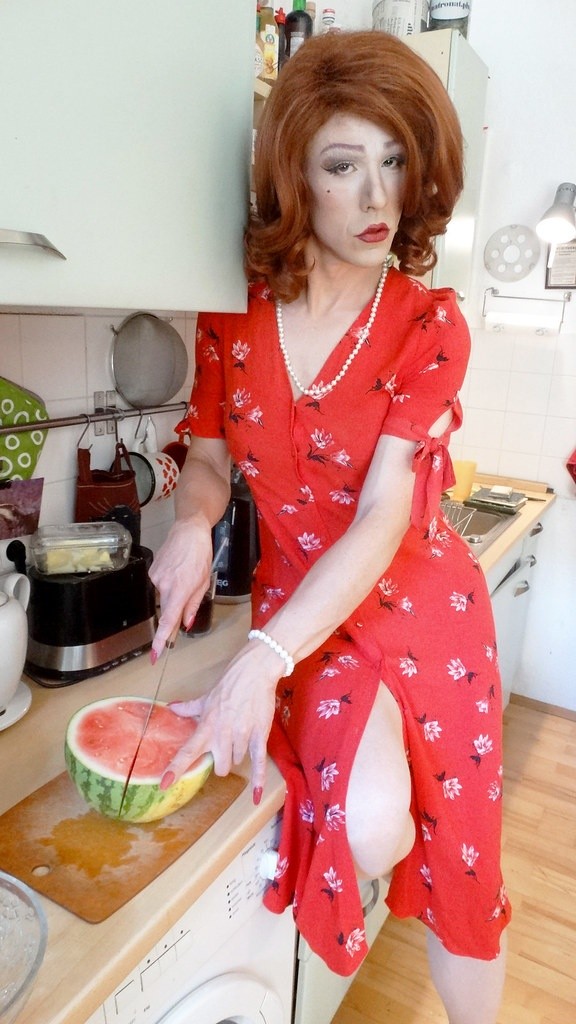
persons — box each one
[148,30,511,1024]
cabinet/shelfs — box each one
[387,29,489,332]
[0,0,258,312]
[484,513,545,713]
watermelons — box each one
[64,696,213,823]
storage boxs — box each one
[28,521,134,576]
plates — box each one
[0,682,32,731]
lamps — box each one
[532,180,576,246]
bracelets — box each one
[248,630,294,678]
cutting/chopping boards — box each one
[0,770,250,924]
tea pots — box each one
[0,574,30,713]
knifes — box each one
[138,607,186,738]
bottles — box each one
[256,0,340,89]
[429,0,472,40]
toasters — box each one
[28,545,155,689]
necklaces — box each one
[276,258,388,395]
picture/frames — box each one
[544,239,576,289]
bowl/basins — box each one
[32,522,132,575]
[0,872,47,1024]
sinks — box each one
[440,505,504,538]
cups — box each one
[109,431,191,511]
[453,460,476,503]
[179,566,218,638]
